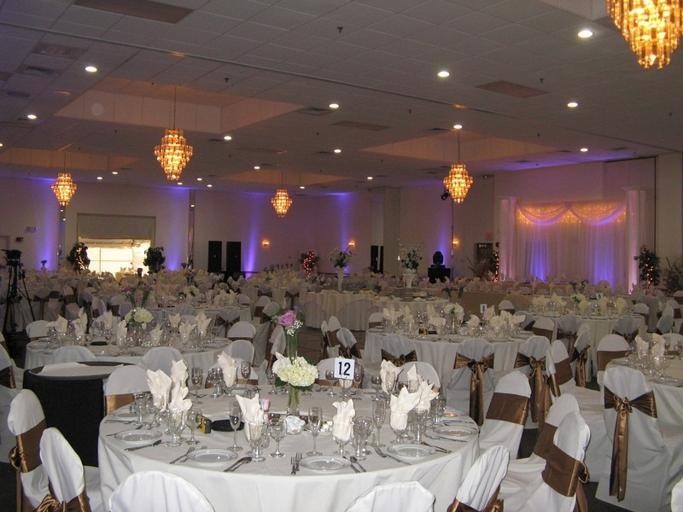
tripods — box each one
[1,264,35,335]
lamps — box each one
[603,0,683,71]
[50,144,78,221]
[442,120,474,205]
[270,150,293,218]
[153,82,194,183]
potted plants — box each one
[397,247,422,288]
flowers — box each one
[328,245,354,271]
[125,306,150,342]
[276,310,304,408]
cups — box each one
[249,422,267,462]
[133,393,147,426]
[371,373,382,400]
[192,367,203,400]
[185,409,203,445]
[145,394,163,427]
[241,361,251,391]
[354,373,361,400]
[660,354,670,383]
[207,371,220,399]
[333,420,353,455]
[308,407,324,458]
[372,399,386,446]
[268,418,287,458]
[165,410,184,446]
[212,368,225,396]
[412,406,428,444]
[266,367,279,394]
[228,407,243,451]
[419,327,428,336]
[388,412,410,446]
[349,415,372,460]
[326,370,337,395]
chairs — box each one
[593,335,627,372]
[0,343,25,388]
[139,347,186,387]
[395,362,440,395]
[226,321,256,347]
[9,388,52,511]
[501,392,580,494]
[26,268,125,322]
[498,412,588,510]
[510,336,551,406]
[602,366,683,512]
[315,358,359,388]
[497,300,514,322]
[530,318,555,340]
[327,315,344,360]
[40,428,93,512]
[547,350,613,483]
[445,445,509,511]
[636,287,682,336]
[611,317,643,344]
[551,338,608,408]
[107,472,215,512]
[227,341,255,370]
[120,286,152,306]
[454,339,493,420]
[343,327,364,360]
[478,370,531,463]
[103,364,153,417]
[129,295,149,309]
[381,335,420,369]
[340,482,433,512]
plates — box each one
[230,384,253,395]
[432,425,477,441]
[206,342,228,349]
[299,456,351,472]
[187,448,236,465]
[114,412,141,421]
[113,429,162,445]
[387,444,434,460]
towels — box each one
[220,288,226,301]
[169,313,180,330]
[180,321,196,344]
[652,333,664,356]
[72,318,88,339]
[151,325,162,344]
[217,352,239,387]
[385,308,391,322]
[493,317,503,334]
[78,307,87,331]
[114,319,127,344]
[579,301,591,313]
[434,318,445,333]
[381,358,402,394]
[198,318,211,338]
[416,379,436,414]
[599,297,607,316]
[196,310,206,323]
[166,387,190,424]
[229,289,235,304]
[617,298,625,315]
[172,361,189,389]
[102,310,114,330]
[340,380,355,390]
[55,315,66,336]
[452,304,466,324]
[484,305,494,322]
[146,367,172,410]
[467,313,478,334]
[406,315,415,328]
[427,302,439,322]
[237,393,264,438]
[333,399,355,441]
[499,310,510,327]
[214,295,220,305]
[664,303,674,316]
[404,305,410,317]
[187,292,194,303]
[511,315,526,333]
[390,387,423,432]
[408,364,421,391]
[534,293,564,310]
[276,351,286,368]
[636,335,648,357]
[205,290,212,302]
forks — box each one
[292,451,303,475]
[376,448,413,467]
[291,456,297,474]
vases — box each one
[329,264,349,290]
[126,323,142,346]
[286,348,307,412]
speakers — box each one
[226,241,241,278]
[208,241,222,273]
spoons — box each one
[124,439,162,451]
[350,456,366,472]
